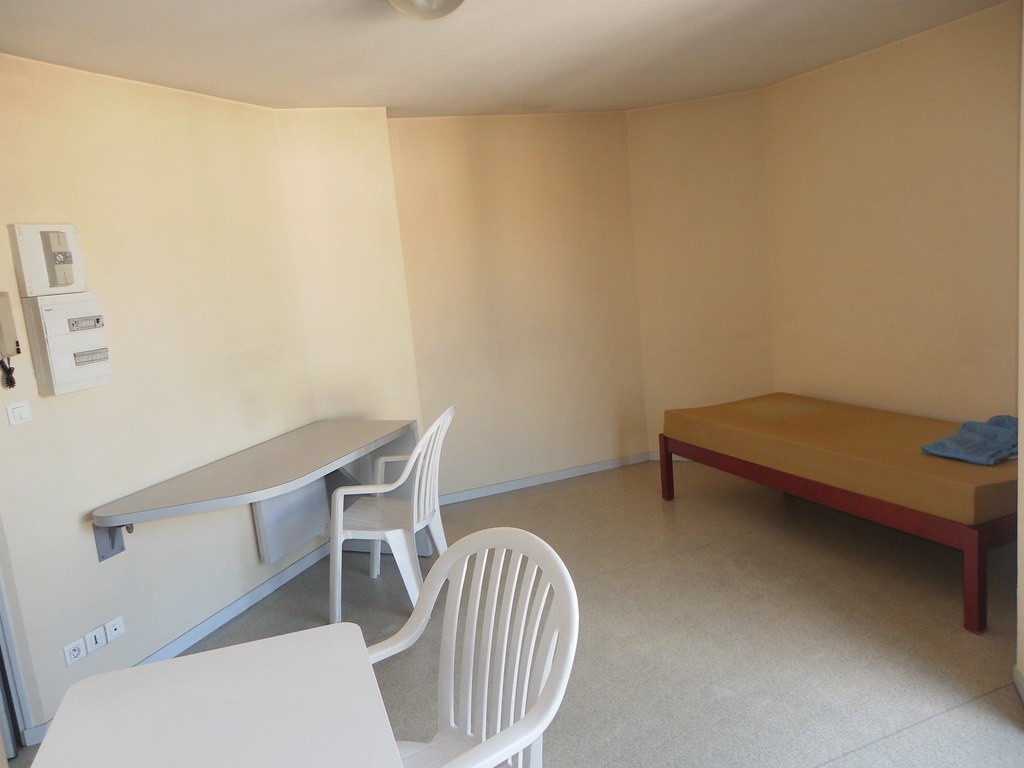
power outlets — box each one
[103,616,127,643]
[84,623,108,655]
[63,636,89,666]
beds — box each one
[658,394,1019,634]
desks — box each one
[91,419,432,562]
[29,621,405,768]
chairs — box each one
[329,404,459,625]
[365,526,578,768]
